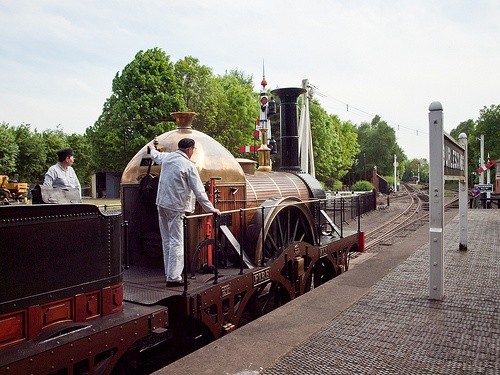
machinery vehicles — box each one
[0,174,30,202]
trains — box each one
[1,61,365,375]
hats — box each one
[57,149,74,162]
[177,138,196,151]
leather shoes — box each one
[166,280,190,287]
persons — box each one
[148,137,221,288]
[41,148,83,205]
[471,184,482,208]
